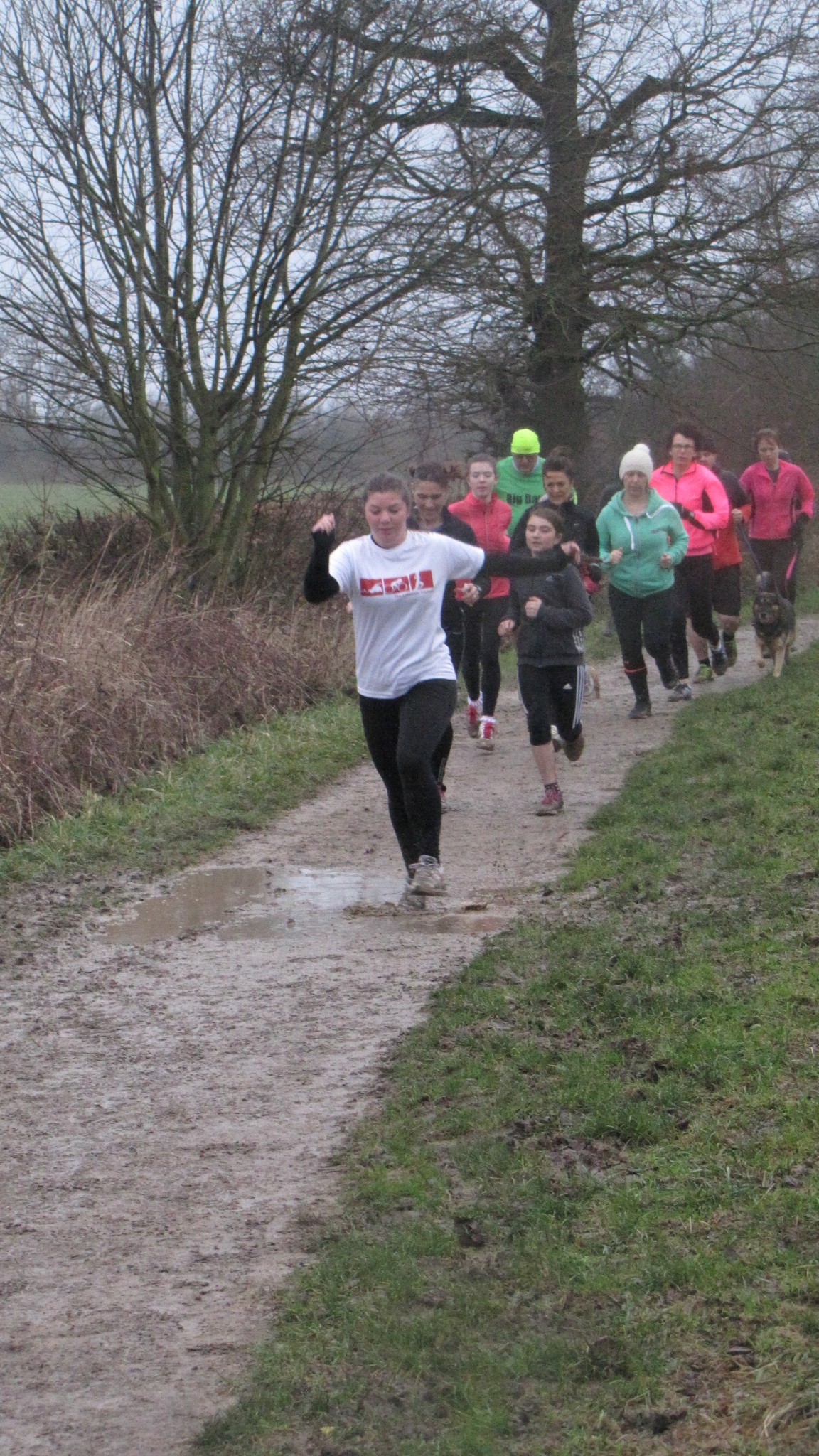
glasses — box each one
[671,444,694,451]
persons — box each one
[303,473,581,916]
[732,429,815,657]
[491,429,578,544]
[504,446,601,751]
[445,453,512,752]
[682,425,752,684]
[402,462,491,811]
[498,507,586,817]
[594,443,689,719]
[647,422,729,704]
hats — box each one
[778,450,792,463]
[510,430,541,454]
[694,436,718,451]
[618,443,655,483]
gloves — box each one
[788,514,810,539]
[668,502,693,521]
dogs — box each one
[751,571,796,678]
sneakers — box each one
[721,634,736,668]
[656,655,677,689]
[710,636,729,676]
[466,705,481,737]
[630,698,653,719]
[439,790,447,814]
[549,724,558,740]
[694,664,714,684]
[762,644,771,657]
[411,854,444,895]
[668,684,691,702]
[478,719,498,749]
[398,877,426,912]
[560,736,587,762]
[536,789,564,816]
